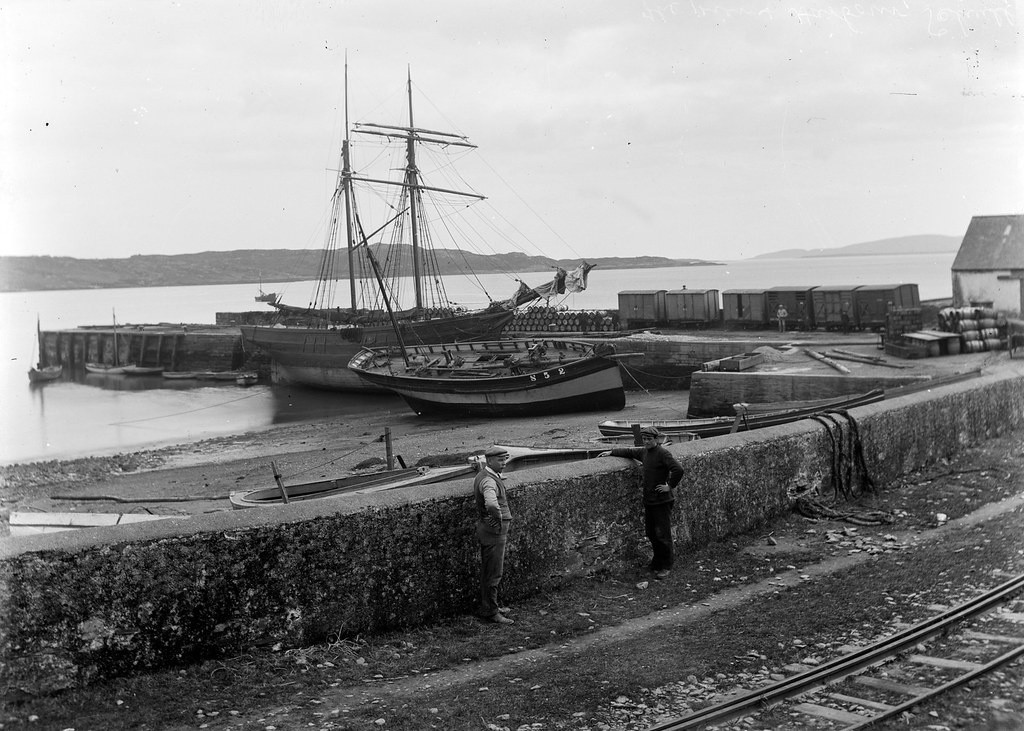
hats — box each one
[640,426,658,437]
[485,446,510,457]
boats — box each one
[192,371,237,379]
[597,388,886,439]
[348,345,626,417]
[465,430,701,474]
[122,366,164,374]
[162,371,197,378]
[237,371,258,387]
[9,511,192,537]
[229,459,483,508]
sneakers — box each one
[641,564,675,578]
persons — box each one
[474,447,514,626]
[777,305,787,332]
[596,426,683,580]
[841,310,849,335]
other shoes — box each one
[480,607,515,625]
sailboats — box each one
[239,45,597,388]
[84,307,135,374]
[253,267,275,301]
[28,312,63,382]
